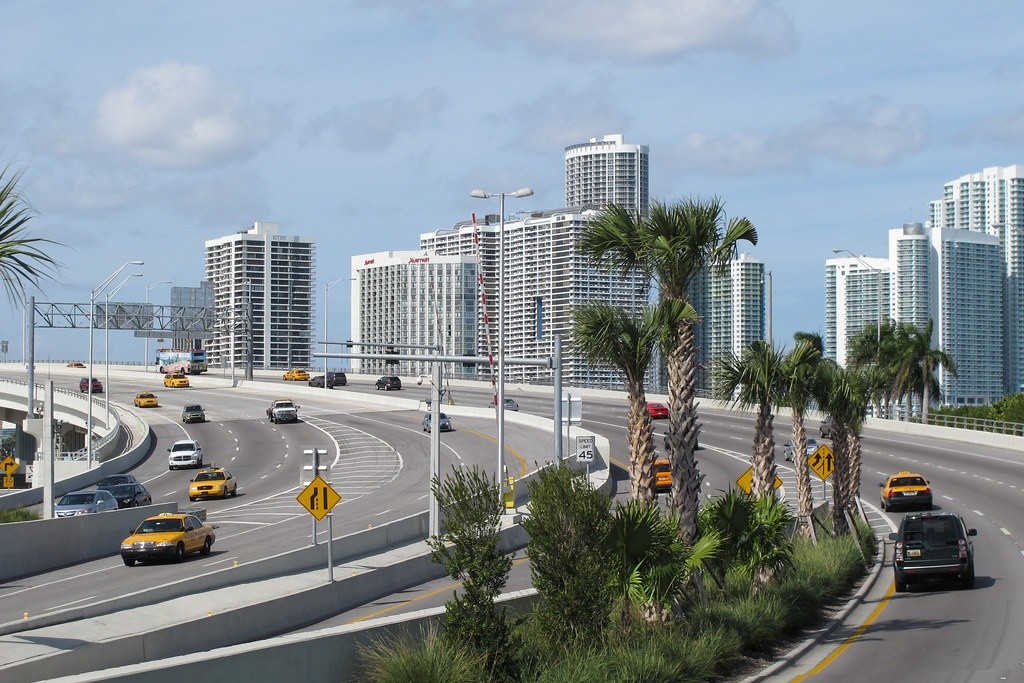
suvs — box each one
[889,512,977,593]
[266,399,301,424]
[167,440,203,471]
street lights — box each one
[470,188,534,511]
[324,278,356,389]
[2,277,25,365]
[832,249,881,419]
[87,261,172,473]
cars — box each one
[783,416,849,464]
[182,404,206,424]
[134,392,159,409]
[421,398,519,433]
[164,372,189,388]
[646,402,699,492]
[67,363,102,394]
[54,468,237,568]
[877,471,933,512]
[283,369,402,391]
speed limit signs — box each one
[576,436,596,463]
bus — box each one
[155,348,207,375]
